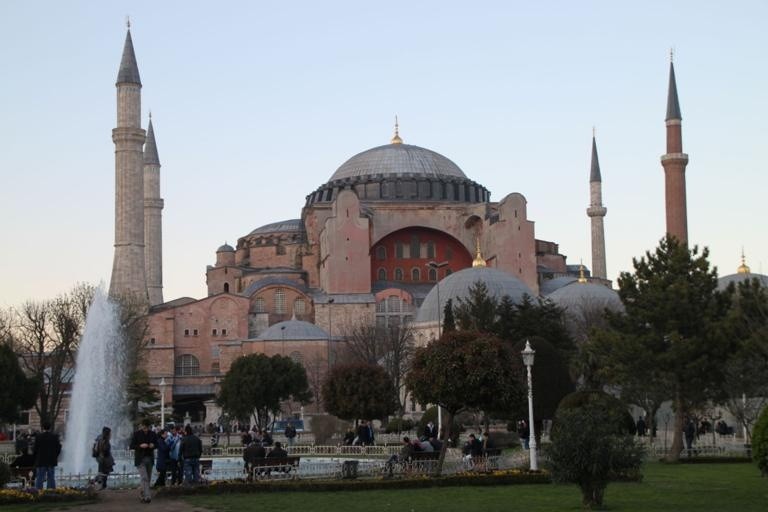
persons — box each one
[633,410,736,460]
[32,421,62,489]
[341,412,540,479]
[9,431,35,487]
[130,415,300,505]
[87,427,115,490]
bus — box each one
[0,423,11,442]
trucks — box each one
[262,419,304,434]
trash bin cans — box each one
[342,461,359,479]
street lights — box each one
[740,373,748,445]
[521,340,538,470]
[159,377,167,431]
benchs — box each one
[463,448,501,457]
[244,456,300,480]
[406,451,439,460]
[8,466,36,488]
[157,460,213,485]
